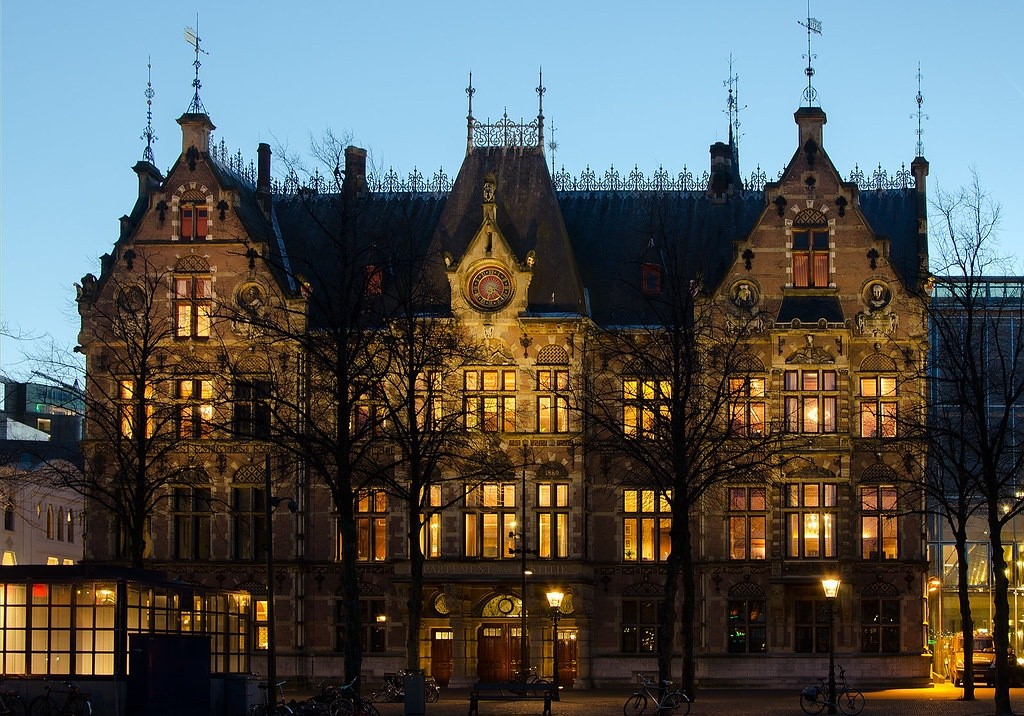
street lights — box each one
[820,570,842,715]
[509,469,537,702]
[545,584,566,701]
[264,454,298,714]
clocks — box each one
[460,257,516,315]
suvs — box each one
[942,631,995,686]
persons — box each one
[1007,646,1017,668]
[870,285,886,306]
[735,285,753,306]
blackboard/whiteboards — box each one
[124,631,214,715]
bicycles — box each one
[623,670,690,716]
[373,666,440,704]
[29,674,93,716]
[251,675,381,716]
[499,666,552,698]
[0,675,28,716]
[800,664,865,715]
